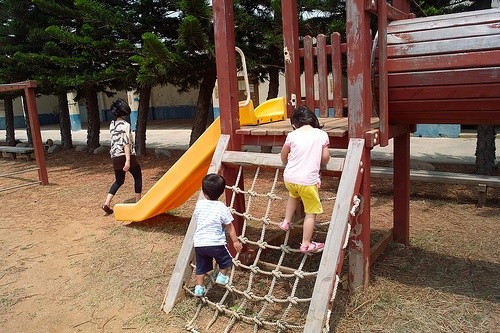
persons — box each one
[279,105,331,253]
[102,98,142,213]
[191,173,243,297]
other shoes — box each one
[278,219,290,230]
[299,241,325,252]
[102,205,113,214]
[215,272,230,286]
[194,285,206,296]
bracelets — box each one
[126,159,131,161]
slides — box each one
[113,96,286,222]
